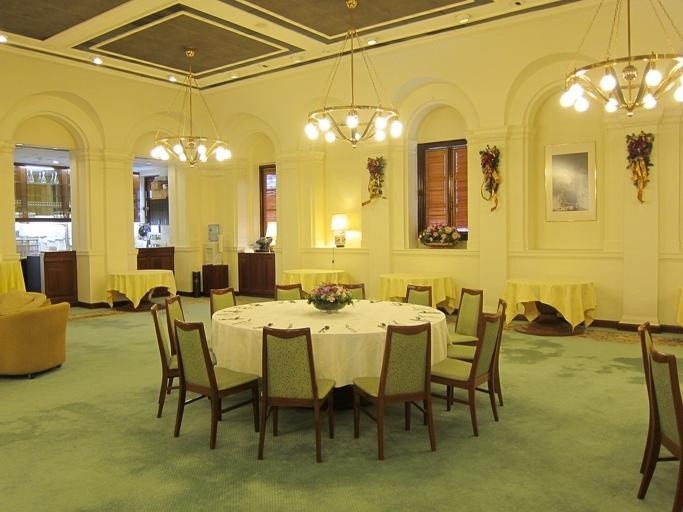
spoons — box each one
[217,296,441,333]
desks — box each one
[211,299,453,410]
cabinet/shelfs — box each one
[201,263,228,297]
[143,175,169,226]
[237,251,277,299]
[12,163,70,223]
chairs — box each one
[636,343,681,511]
[174,319,260,448]
[0,291,70,377]
[353,323,436,460]
[274,284,305,301]
[258,327,336,463]
[634,319,680,475]
[166,296,218,394]
[151,304,218,418]
[405,285,432,307]
[448,288,484,346]
[343,284,365,299]
[447,299,504,407]
[210,287,235,318]
[423,311,501,436]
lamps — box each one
[149,47,235,167]
[330,214,349,248]
[298,2,408,151]
[554,1,683,117]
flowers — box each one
[415,222,461,243]
[623,130,655,206]
[477,145,503,211]
[307,285,355,314]
[362,156,387,205]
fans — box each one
[137,222,151,240]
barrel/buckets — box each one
[208,224,219,241]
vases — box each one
[425,240,458,248]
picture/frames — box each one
[542,141,599,224]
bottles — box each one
[50,172,54,181]
[53,171,59,184]
[38,172,41,180]
[29,170,34,185]
[40,172,46,183]
[26,171,29,183]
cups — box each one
[16,199,64,218]
